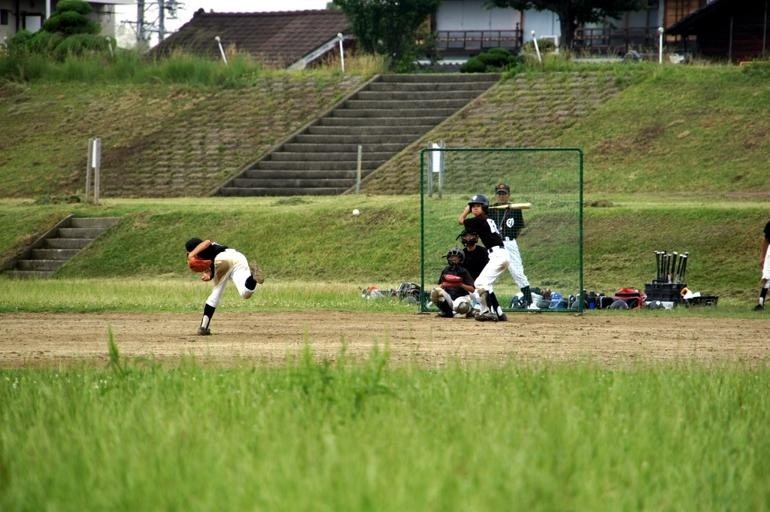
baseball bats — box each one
[653,250,688,285]
[487,203,530,209]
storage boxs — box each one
[645,282,687,305]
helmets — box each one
[468,194,489,213]
[461,230,479,245]
[445,247,466,264]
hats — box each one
[495,184,510,194]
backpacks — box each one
[397,282,427,305]
[615,287,648,308]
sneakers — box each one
[754,304,764,310]
[478,311,499,321]
[498,312,506,321]
[198,326,211,335]
[249,259,264,284]
[466,308,480,318]
[439,311,454,317]
[513,295,532,309]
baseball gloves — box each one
[442,274,462,287]
[188,256,212,272]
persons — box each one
[428,248,475,319]
[487,182,542,311]
[456,228,494,307]
[458,195,511,321]
[751,220,770,311]
[183,236,265,337]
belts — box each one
[490,245,504,252]
[502,237,516,240]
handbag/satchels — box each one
[569,290,614,308]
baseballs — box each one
[353,209,360,215]
